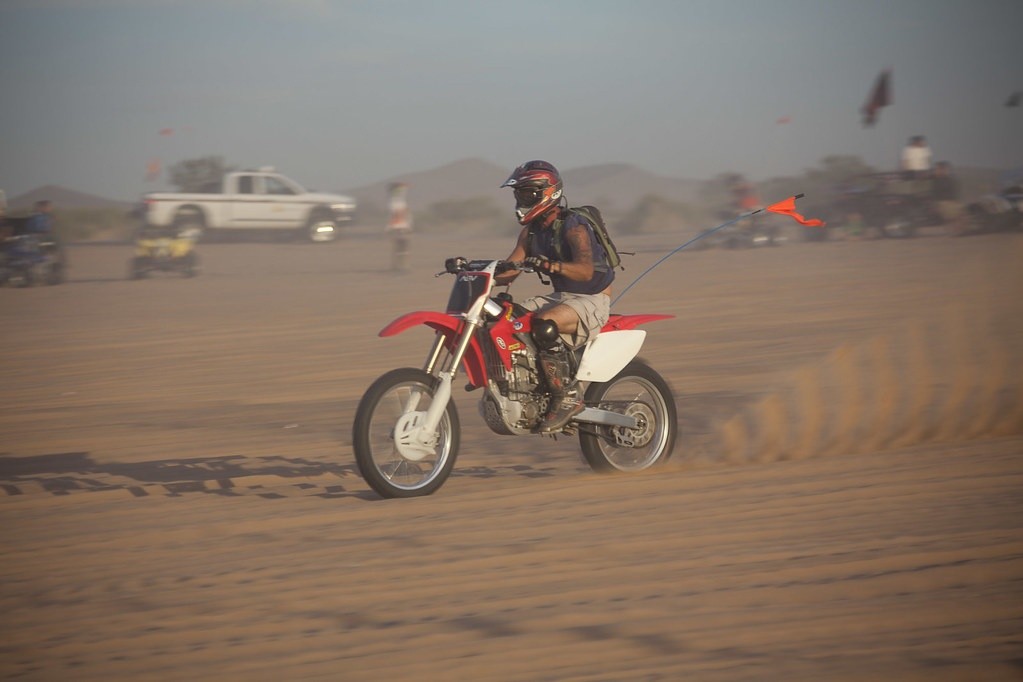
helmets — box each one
[498,160,565,227]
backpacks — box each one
[527,206,637,275]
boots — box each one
[537,349,587,436]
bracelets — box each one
[556,261,562,275]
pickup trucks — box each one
[140,167,357,245]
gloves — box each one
[522,254,551,277]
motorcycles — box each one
[352,254,679,500]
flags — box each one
[768,197,826,227]
[859,70,894,110]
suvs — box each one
[0,211,66,288]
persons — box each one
[28,202,55,234]
[727,174,756,212]
[901,134,933,172]
[386,181,416,275]
[445,160,615,432]
